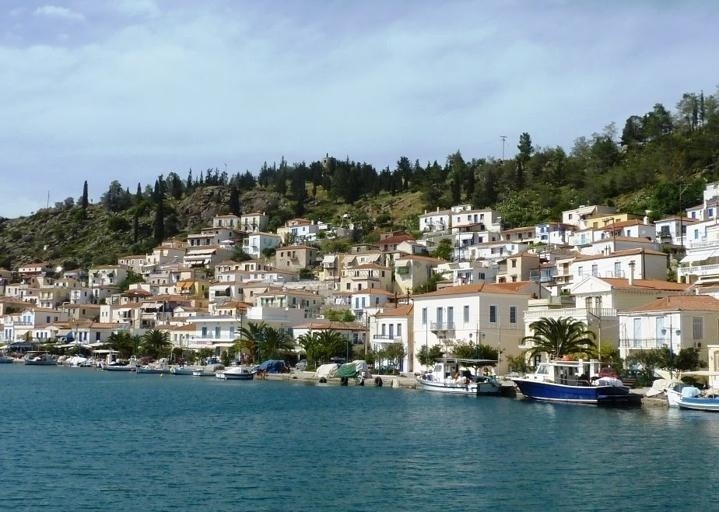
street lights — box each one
[468,323,486,376]
[661,316,681,380]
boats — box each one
[0,345,256,381]
[412,356,502,397]
[665,384,719,414]
[504,359,644,406]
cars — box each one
[295,358,309,369]
[620,369,646,385]
